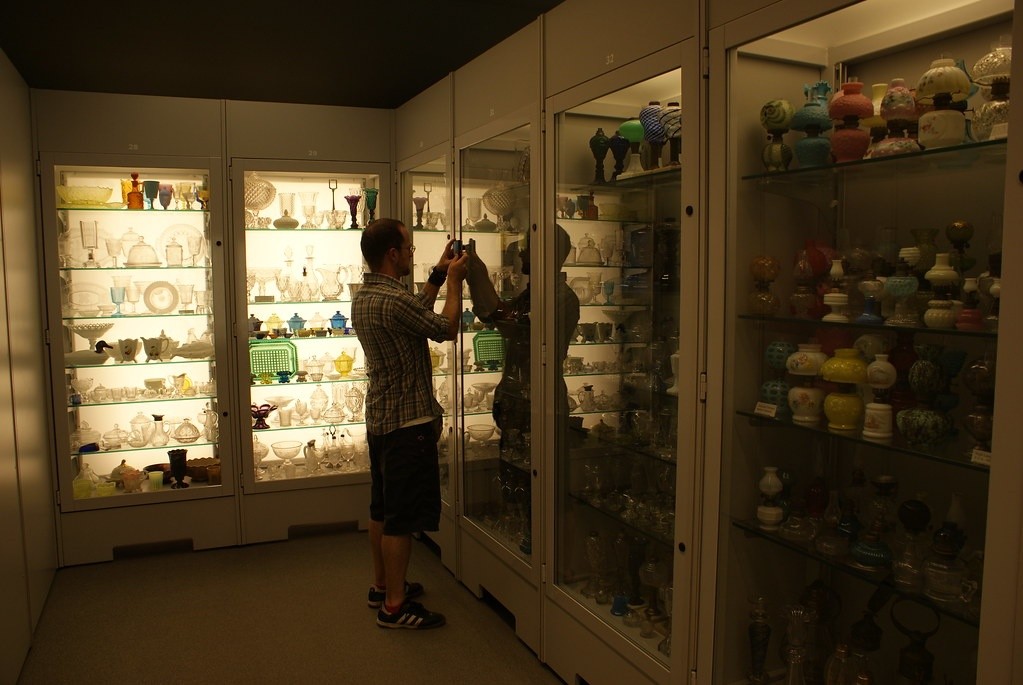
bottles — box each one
[746,45,979,685]
[127,181,143,208]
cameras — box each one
[453,240,466,258]
[462,238,476,256]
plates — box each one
[59,201,129,209]
[143,282,179,315]
[123,262,162,267]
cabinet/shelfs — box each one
[455,15,542,667]
[705,0,1023,685]
[394,72,458,584]
[542,0,705,685]
[30,87,243,566]
[560,185,660,444]
[225,97,393,547]
[403,172,528,468]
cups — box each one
[194,291,205,304]
[165,238,182,266]
[119,337,170,363]
[121,227,139,255]
[461,465,674,658]
[249,312,356,337]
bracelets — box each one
[428,266,447,287]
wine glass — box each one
[110,287,140,315]
[177,284,194,313]
[143,181,209,210]
[187,235,202,267]
[104,238,122,267]
[412,194,680,468]
[246,245,369,301]
[298,186,378,229]
[80,219,100,268]
[67,352,370,500]
[327,211,347,229]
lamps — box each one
[913,54,972,150]
[868,78,921,159]
[924,254,965,329]
[861,354,899,439]
[822,260,851,324]
[758,341,800,414]
[751,254,781,316]
[756,465,785,533]
[968,45,1011,142]
[818,347,867,432]
[828,81,875,165]
[790,88,832,170]
[785,344,829,424]
[758,99,795,174]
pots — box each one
[105,343,141,361]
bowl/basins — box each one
[79,310,98,316]
[57,184,113,204]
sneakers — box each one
[368,581,446,630]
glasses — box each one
[396,244,417,253]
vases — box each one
[609,130,631,180]
[616,117,645,180]
[660,102,682,165]
[639,101,669,169]
[589,127,610,183]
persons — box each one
[350,217,468,629]
[467,222,581,555]
